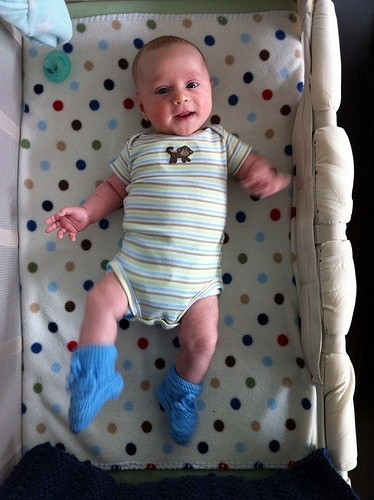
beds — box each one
[0,0,359,500]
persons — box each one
[44,35,292,446]
